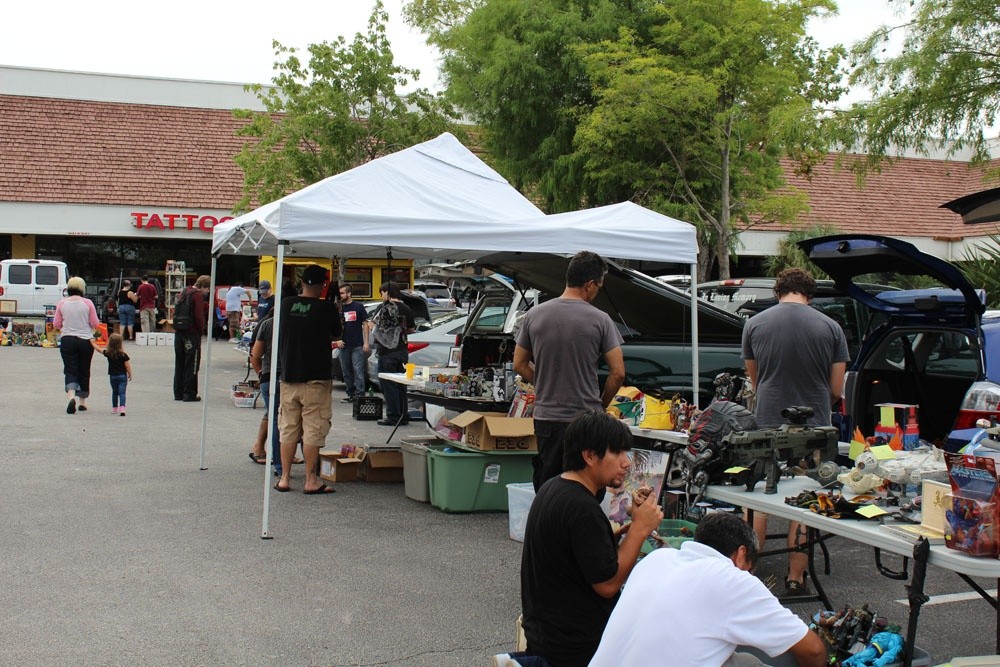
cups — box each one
[406,363,415,379]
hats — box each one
[259,280,270,294]
[302,264,327,283]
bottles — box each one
[368,385,373,397]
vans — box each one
[0,259,71,319]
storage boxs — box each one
[352,396,383,421]
[427,445,538,513]
[449,410,538,451]
[399,437,444,502]
[319,451,362,482]
[640,518,698,555]
[354,447,405,482]
[136,319,175,346]
[506,482,536,543]
[231,390,265,408]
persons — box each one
[842,623,903,667]
[335,284,369,402]
[136,274,158,332]
[53,277,99,414]
[587,513,828,667]
[119,280,137,340]
[90,333,133,416]
[226,281,253,343]
[249,307,304,479]
[368,282,416,426]
[274,265,344,495]
[173,275,211,401]
[494,409,664,667]
[244,280,275,323]
[513,251,626,505]
[740,269,852,594]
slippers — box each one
[303,484,335,493]
[292,457,304,464]
[274,481,290,491]
[249,453,273,465]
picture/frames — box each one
[448,347,460,368]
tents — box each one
[201,132,700,540]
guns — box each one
[720,405,840,495]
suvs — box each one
[98,276,166,332]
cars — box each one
[330,262,542,395]
[799,235,1000,454]
[213,285,259,327]
[477,251,914,404]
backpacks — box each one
[173,287,199,330]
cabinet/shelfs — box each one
[166,261,186,319]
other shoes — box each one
[67,399,76,414]
[377,419,408,426]
[340,398,349,402]
[183,394,201,401]
[787,570,810,595]
[174,395,182,400]
[506,659,521,667]
[113,407,118,414]
[489,653,511,667]
[78,405,87,410]
[119,406,125,416]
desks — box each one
[628,424,1000,667]
[378,373,511,445]
